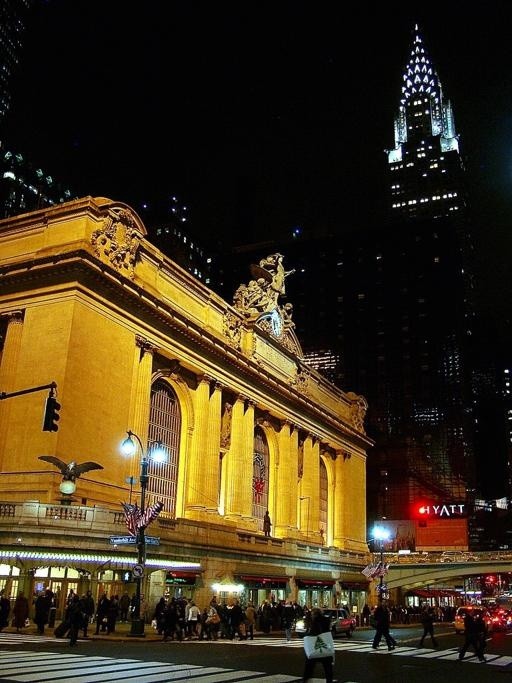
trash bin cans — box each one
[256,615,263,632]
[369,615,375,625]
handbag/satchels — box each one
[197,610,203,623]
[128,602,135,612]
[302,631,336,660]
[24,618,30,627]
[210,613,221,624]
[151,617,159,630]
[103,618,109,623]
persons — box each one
[262,511,272,537]
[232,252,297,330]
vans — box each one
[489,549,512,561]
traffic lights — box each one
[122,571,132,581]
[42,397,61,433]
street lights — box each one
[121,428,167,638]
[370,524,391,603]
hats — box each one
[41,591,45,596]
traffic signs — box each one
[144,536,160,545]
[110,535,136,544]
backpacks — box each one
[235,607,244,622]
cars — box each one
[463,552,474,556]
[455,592,511,634]
[435,550,479,563]
[375,551,432,563]
[293,607,357,639]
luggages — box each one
[54,622,74,637]
